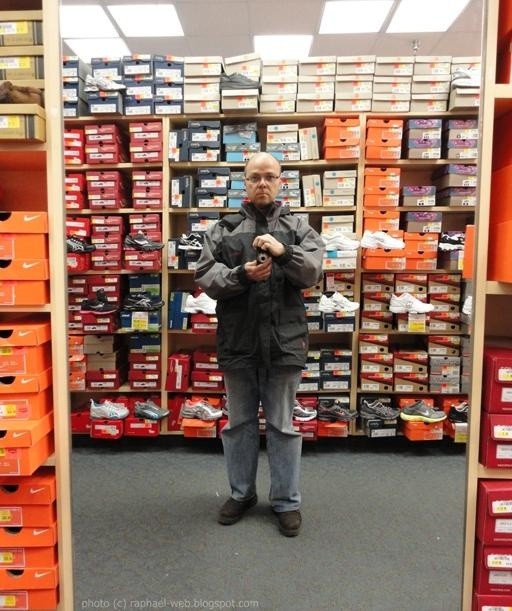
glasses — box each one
[243,173,281,183]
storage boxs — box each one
[470,346,511,611]
[0,11,60,611]
[62,51,483,444]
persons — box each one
[192,149,325,539]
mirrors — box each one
[53,0,488,611]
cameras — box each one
[256,246,269,265]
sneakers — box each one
[83,74,126,93]
[178,230,206,250]
[122,291,165,312]
[180,399,223,421]
[450,68,481,87]
[184,291,217,314]
[389,290,435,314]
[438,232,465,252]
[318,399,360,422]
[89,397,129,420]
[449,400,468,423]
[400,399,448,423]
[65,233,96,253]
[222,400,229,415]
[318,290,361,314]
[123,229,166,252]
[360,398,400,421]
[361,229,407,250]
[134,399,170,421]
[220,72,261,90]
[218,493,259,525]
[462,294,472,314]
[292,399,317,421]
[79,287,120,315]
[276,509,302,537]
[321,232,360,252]
